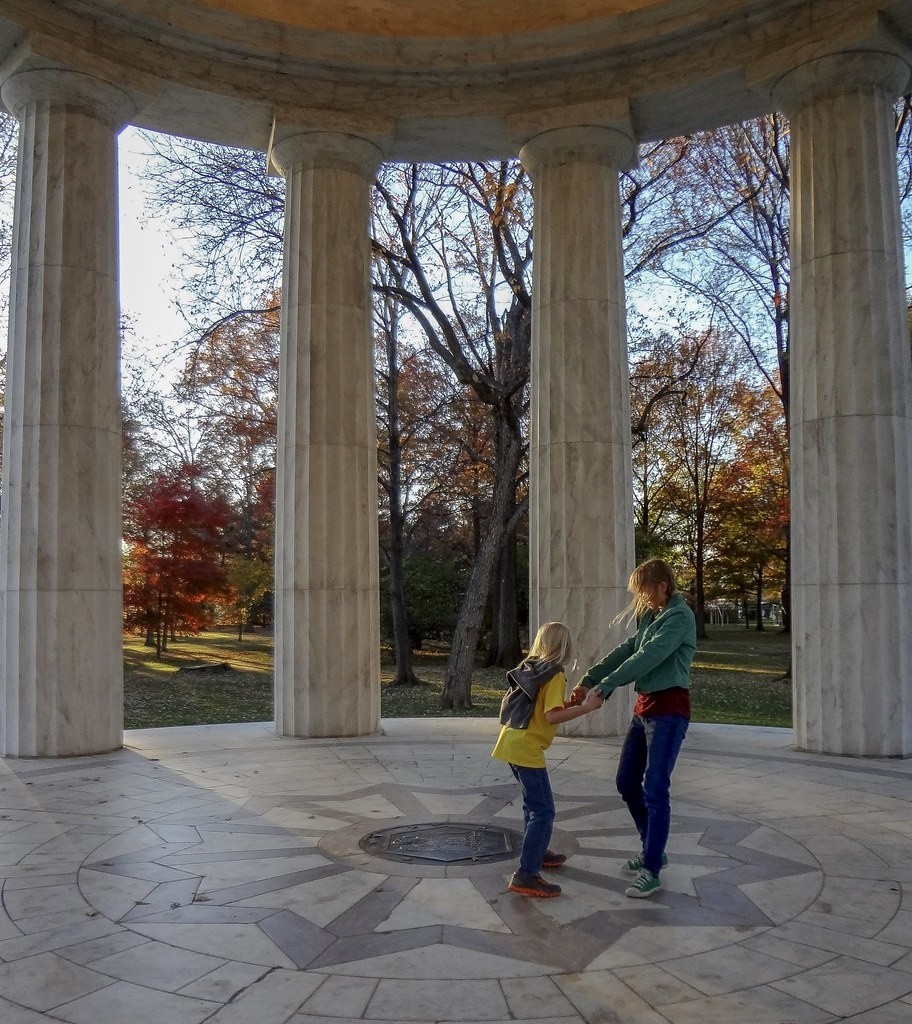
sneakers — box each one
[620,850,669,874]
[507,871,562,896]
[545,851,567,867]
[625,872,660,897]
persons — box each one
[570,558,695,898]
[488,621,603,898]
